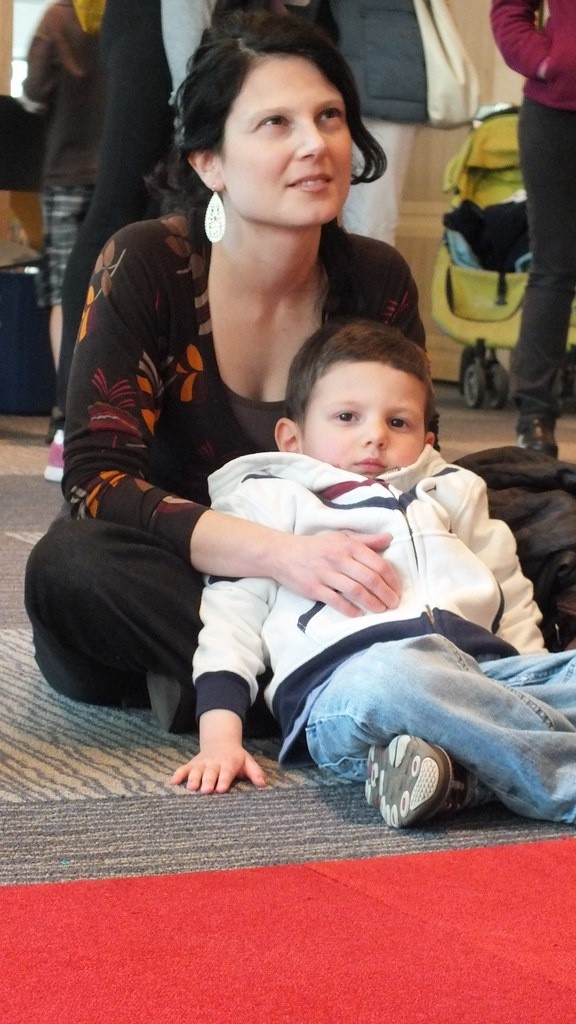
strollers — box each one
[432,103,576,416]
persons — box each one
[41,0,175,482]
[490,1,574,461]
[160,1,484,419]
[24,9,445,733]
[25,1,134,450]
[171,315,576,828]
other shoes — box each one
[365,734,471,830]
[44,430,65,483]
[517,419,559,458]
